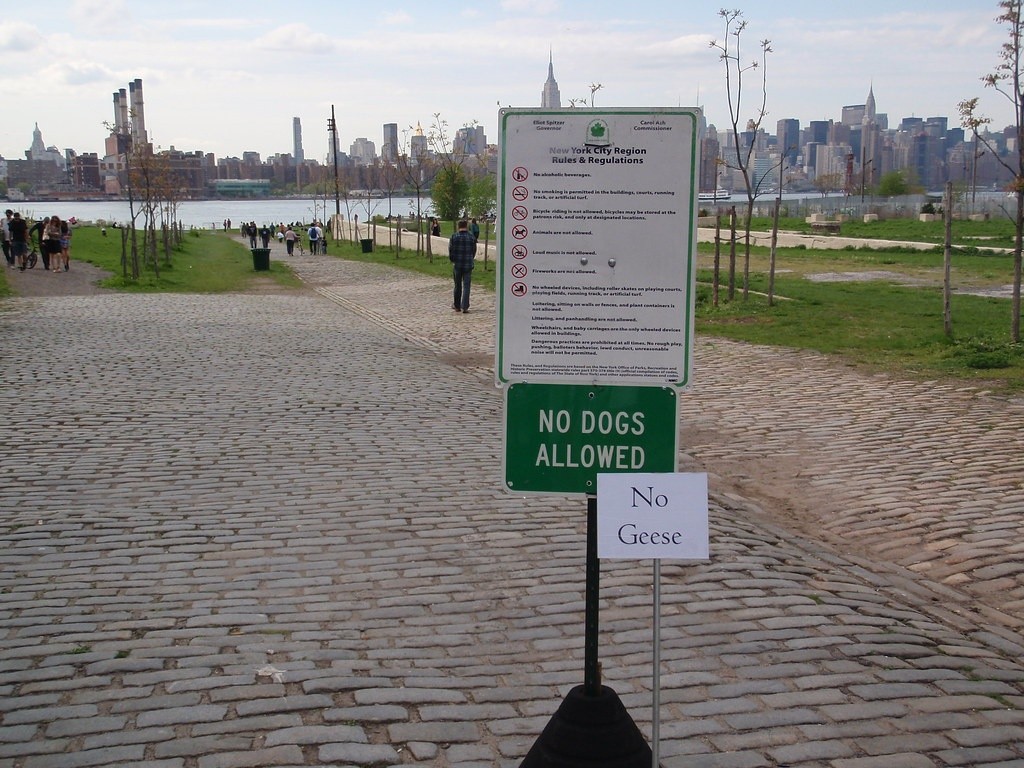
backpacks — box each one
[310,228,318,238]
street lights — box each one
[328,118,340,215]
[779,167,788,203]
[861,159,872,204]
[972,151,985,202]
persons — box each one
[470,218,479,243]
[0,210,78,273]
[431,219,441,237]
[112,222,118,228]
[223,219,327,256]
[449,221,478,313]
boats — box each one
[698,190,731,200]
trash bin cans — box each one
[360,239,373,253]
[251,249,271,270]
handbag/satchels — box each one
[42,224,51,241]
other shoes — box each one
[463,308,469,313]
[452,304,461,312]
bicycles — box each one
[296,237,306,256]
[318,236,323,256]
[26,239,41,270]
